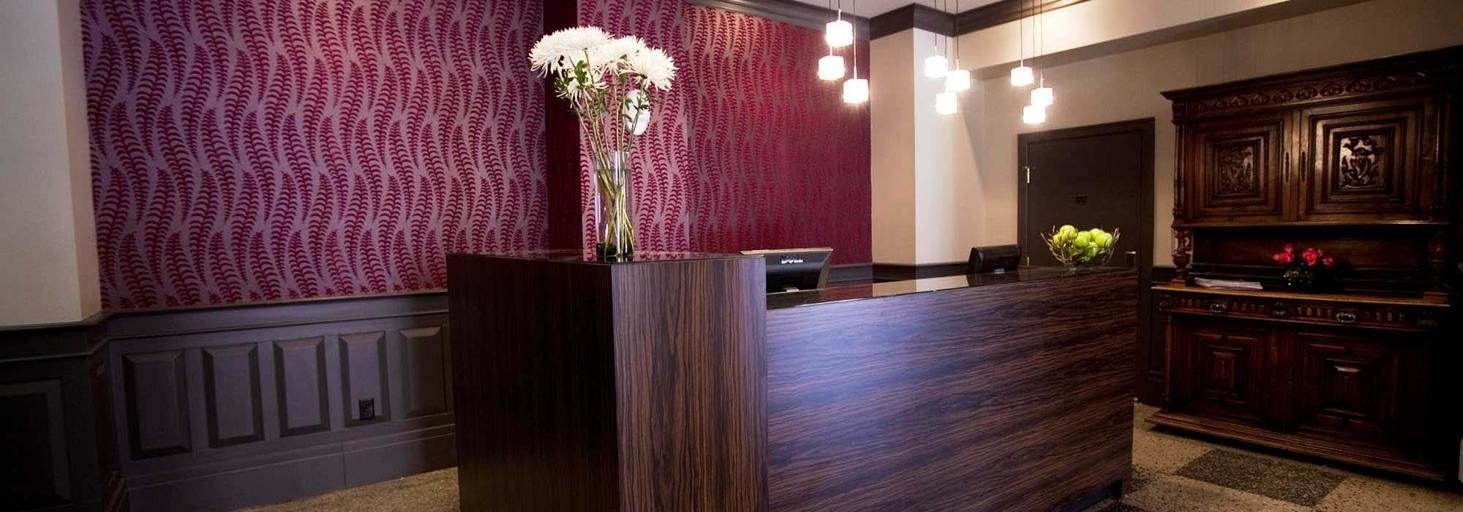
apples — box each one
[1051,225,1113,263]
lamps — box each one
[815,0,1052,126]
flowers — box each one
[526,26,681,254]
[1271,241,1335,271]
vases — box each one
[592,150,635,262]
[1284,260,1315,294]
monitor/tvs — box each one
[739,246,835,294]
[966,243,1022,273]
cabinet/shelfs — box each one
[1171,295,1291,434]
[1296,99,1425,222]
[1427,87,1459,234]
[1406,311,1461,453]
[1294,305,1408,444]
[1183,106,1297,223]
